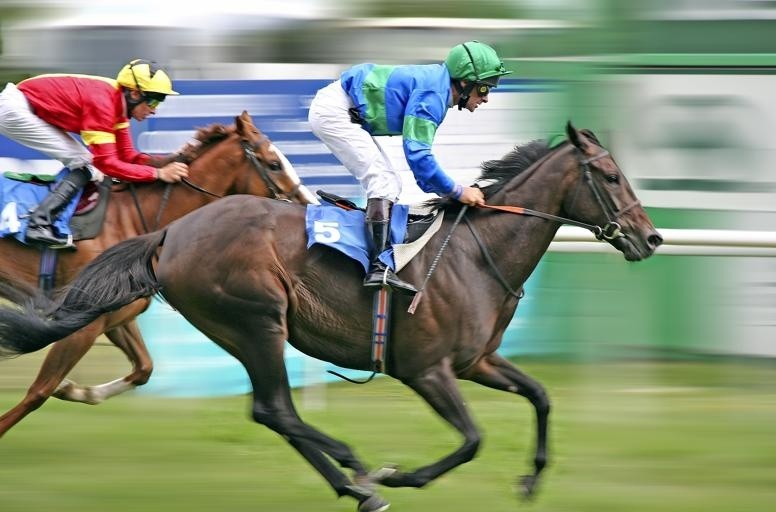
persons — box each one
[0,56,189,250]
[308,41,502,294]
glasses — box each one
[473,84,490,96]
[144,96,160,109]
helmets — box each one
[445,39,515,81]
[117,58,181,95]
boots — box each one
[362,197,418,296]
[25,166,92,252]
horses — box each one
[1,107,321,432]
[0,116,664,512]
